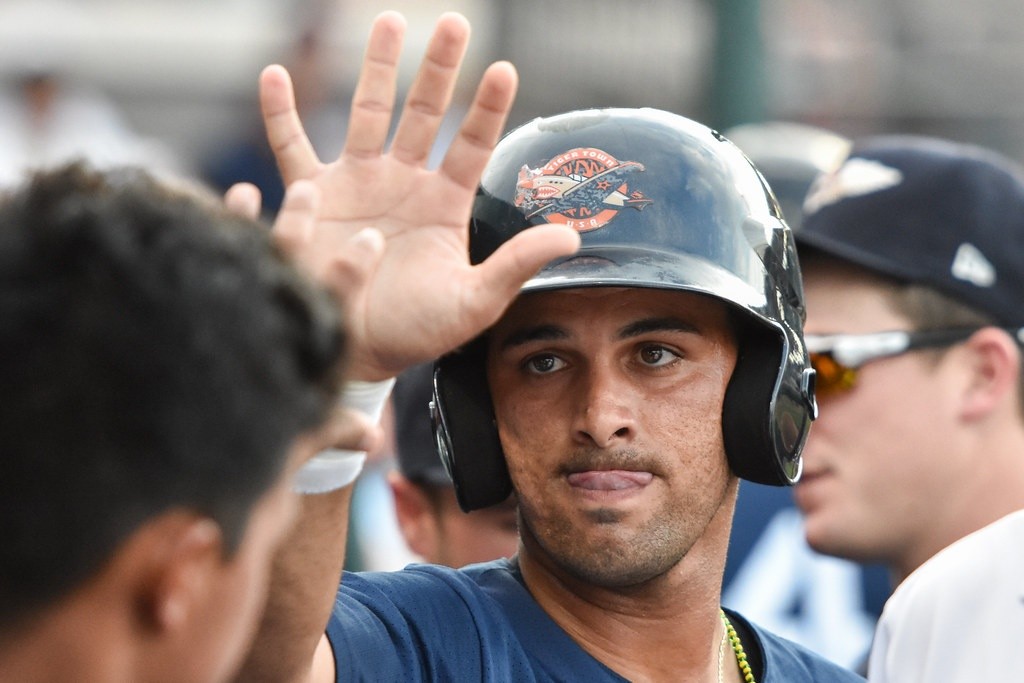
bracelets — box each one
[291,374,394,495]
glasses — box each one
[804,325,1024,408]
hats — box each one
[391,364,451,486]
[792,134,1024,326]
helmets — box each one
[726,123,847,217]
[429,108,818,512]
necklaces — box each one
[717,624,728,683]
[719,607,755,683]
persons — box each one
[0,151,385,683]
[2,0,900,674]
[255,12,870,683]
[788,130,1024,683]
